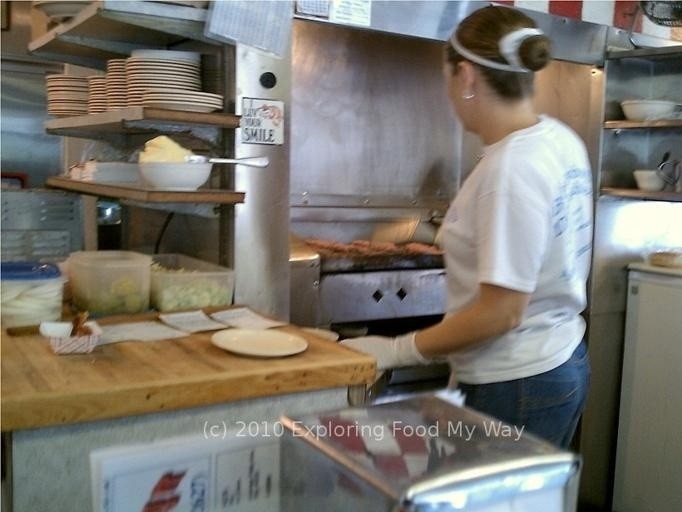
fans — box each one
[626,1,681,53]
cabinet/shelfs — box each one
[598,45,681,204]
[31,1,244,204]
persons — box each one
[338,7,593,449]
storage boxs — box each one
[148,254,235,310]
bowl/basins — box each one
[633,169,668,192]
[136,160,213,192]
[618,99,682,121]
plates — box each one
[33,2,96,22]
[43,49,225,118]
[214,330,312,357]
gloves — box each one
[341,331,432,372]
